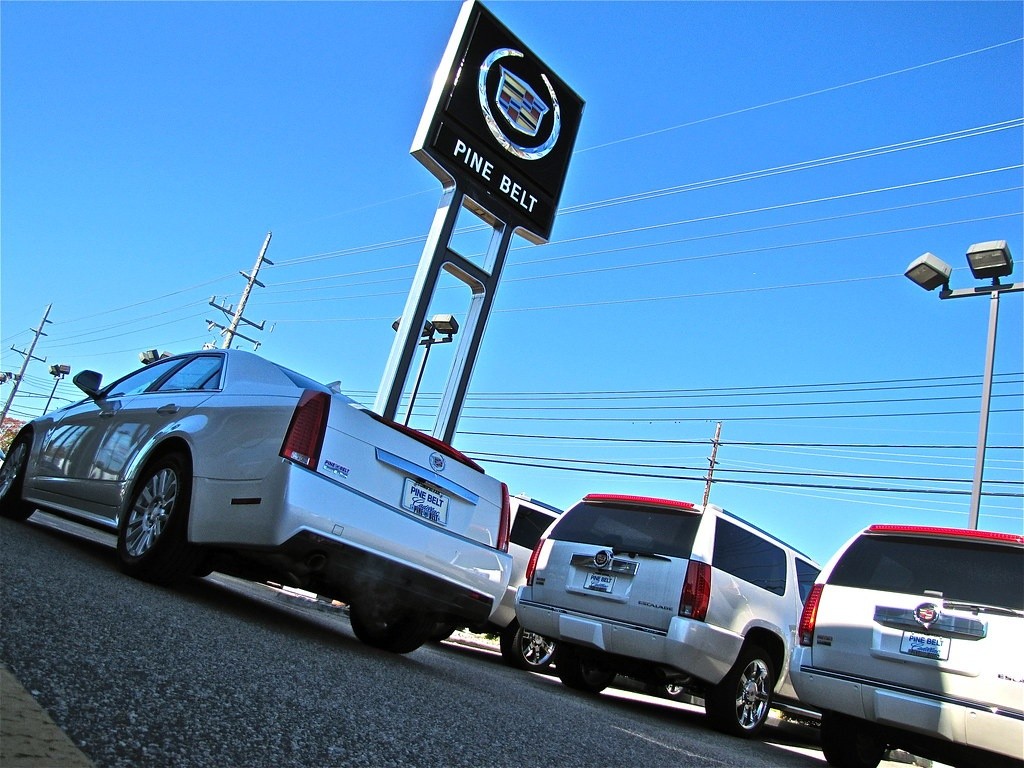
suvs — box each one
[514,494,823,736]
[429,492,565,674]
[791,522,1024,768]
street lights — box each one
[42,364,70,416]
[392,314,459,427]
[905,240,1024,531]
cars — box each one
[0,349,515,653]
